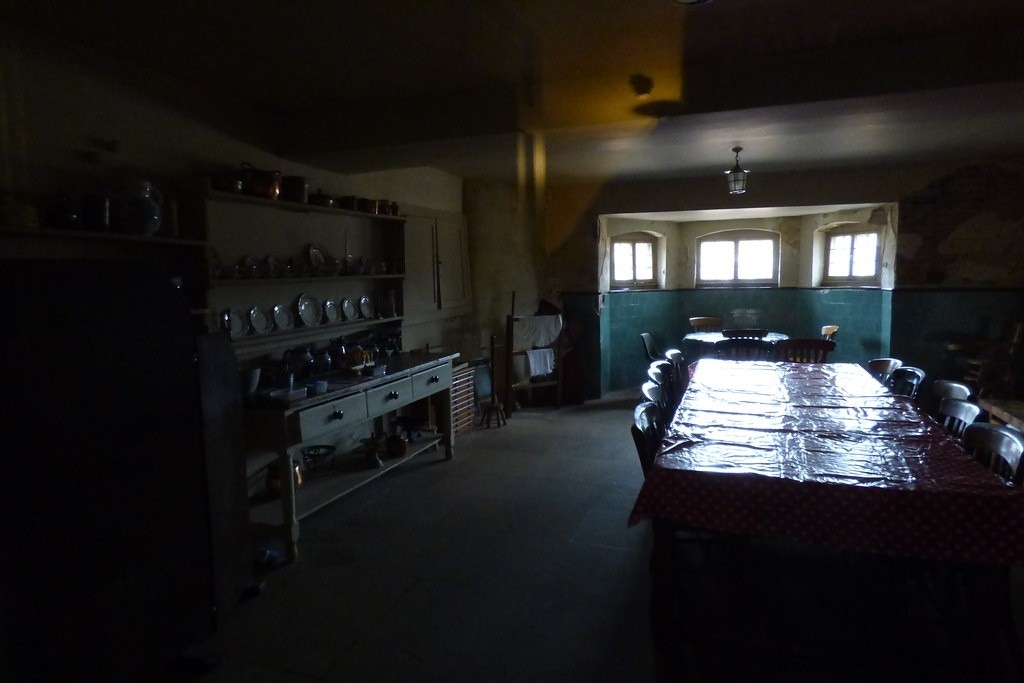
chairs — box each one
[689,315,727,333]
[640,333,666,365]
[932,392,993,450]
[912,379,973,428]
[821,325,838,362]
[865,357,903,388]
[770,333,835,364]
[712,338,772,362]
[963,419,1024,486]
[886,366,925,404]
[627,348,761,601]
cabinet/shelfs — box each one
[177,184,411,357]
[392,204,468,330]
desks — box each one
[682,330,791,362]
[243,344,462,562]
[627,356,1022,632]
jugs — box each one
[118,181,163,237]
[282,335,380,378]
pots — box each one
[239,166,281,198]
[284,174,312,203]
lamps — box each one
[726,144,747,195]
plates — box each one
[225,308,249,339]
[298,293,322,326]
[273,304,295,331]
[324,299,341,322]
[310,247,325,266]
[250,306,272,334]
[360,295,375,318]
[343,298,359,320]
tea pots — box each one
[383,432,408,456]
